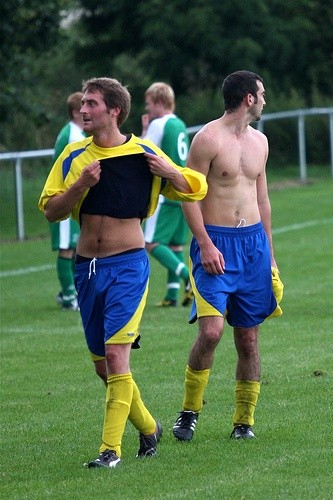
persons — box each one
[38,77,208,468]
[140,81,195,310]
[173,71,284,442]
[49,92,89,311]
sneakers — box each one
[229,424,257,441]
[155,298,177,306]
[173,410,199,442]
[56,291,81,312]
[136,420,163,459]
[88,448,121,469]
[182,273,194,306]
